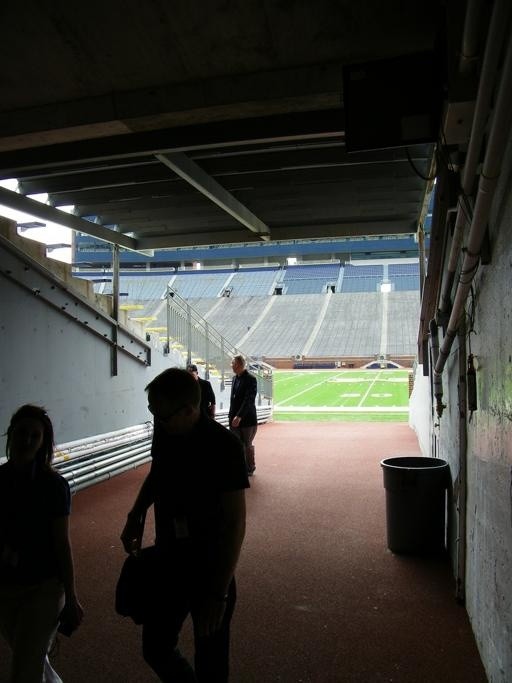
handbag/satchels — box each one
[115,545,180,625]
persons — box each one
[229,355,258,476]
[187,364,216,420]
[0,403,86,682]
[120,367,251,683]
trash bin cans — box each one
[381,456,450,558]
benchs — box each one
[19,221,219,370]
